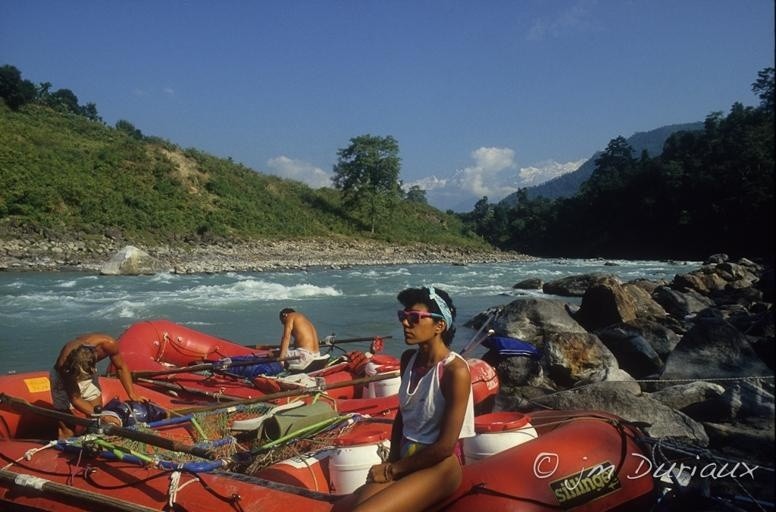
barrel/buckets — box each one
[361,354,401,399]
[329,428,391,496]
[374,363,402,397]
[461,411,539,465]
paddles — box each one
[130,356,300,379]
[0,392,217,461]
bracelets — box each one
[384,462,393,482]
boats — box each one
[0,369,659,511]
[110,317,501,420]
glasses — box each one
[397,309,442,323]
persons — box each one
[47,332,152,442]
[330,284,477,512]
[275,306,322,372]
